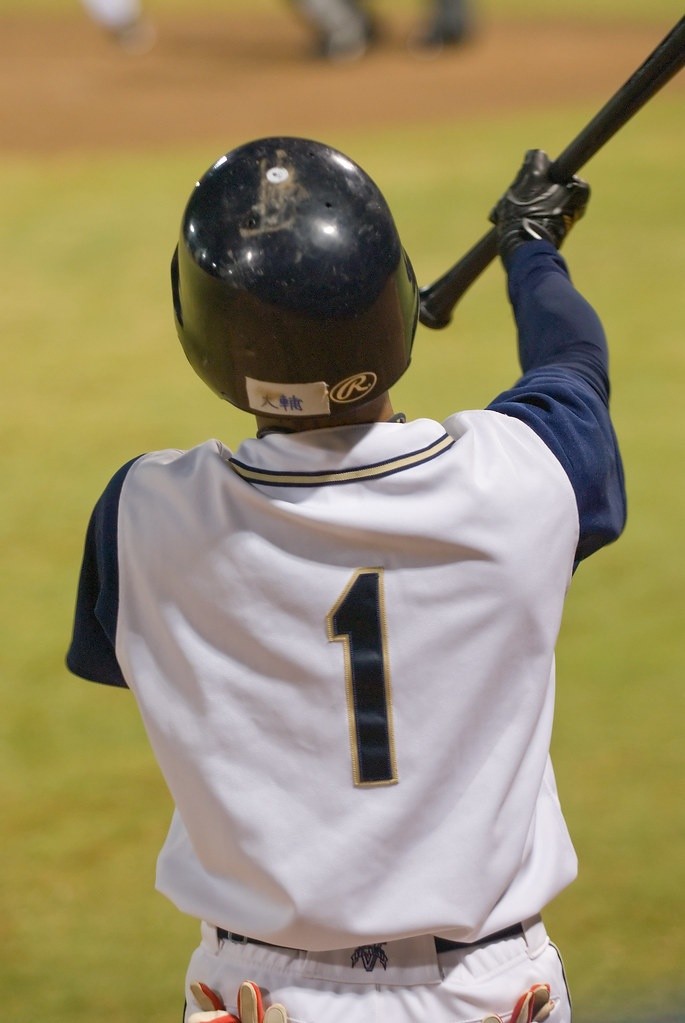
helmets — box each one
[169,136,421,419]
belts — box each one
[217,920,523,954]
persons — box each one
[294,0,461,57]
[65,134,628,1022]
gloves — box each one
[188,980,287,1023]
[483,982,557,1023]
[488,149,591,261]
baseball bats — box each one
[414,16,685,331]
